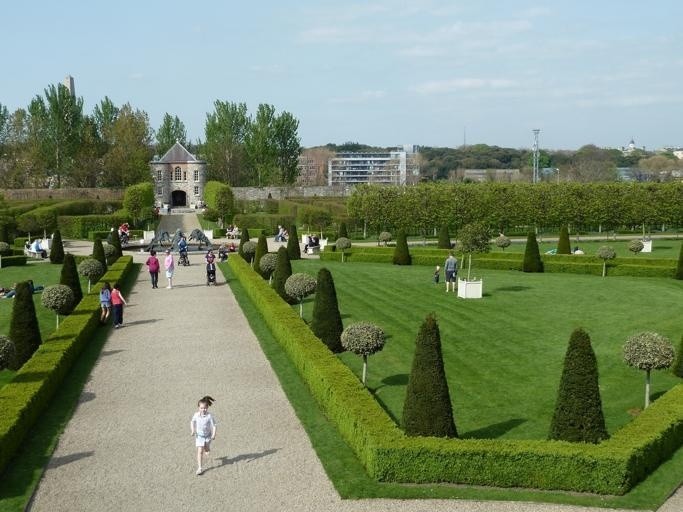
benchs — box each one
[225,230,243,239]
[276,234,287,242]
[305,245,320,255]
[23,244,46,260]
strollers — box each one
[206,262,217,286]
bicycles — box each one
[116,231,128,244]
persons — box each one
[189,398,216,474]
[573,245,584,254]
[443,251,458,292]
[24,238,47,259]
[434,265,439,284]
[97,281,110,324]
[105,216,332,290]
[0,278,44,301]
[109,283,127,329]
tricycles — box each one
[177,250,189,266]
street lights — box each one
[532,129,541,181]
